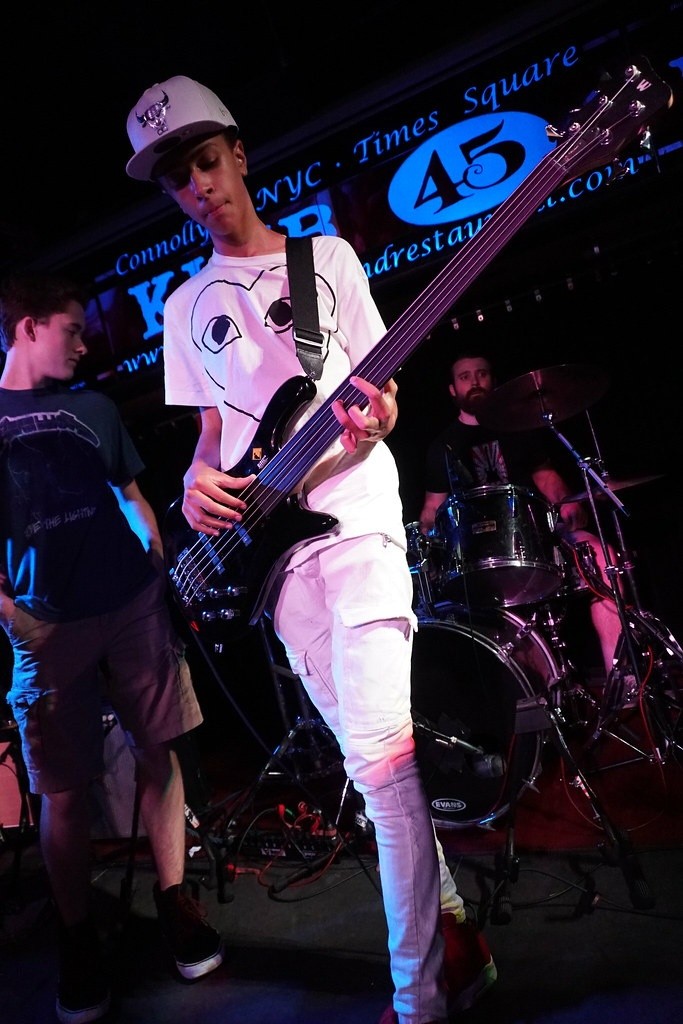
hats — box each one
[125,76,240,182]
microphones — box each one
[444,444,473,484]
[446,737,507,781]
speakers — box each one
[0,707,152,841]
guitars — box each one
[156,54,677,642]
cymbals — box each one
[556,474,662,506]
[487,363,591,432]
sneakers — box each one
[152,879,223,979]
[55,914,111,1024]
[381,910,500,1024]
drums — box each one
[433,480,562,609]
[411,602,561,835]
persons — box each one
[121,76,498,1024]
[0,275,220,1024]
[416,340,639,705]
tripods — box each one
[535,392,683,780]
[224,667,490,898]
